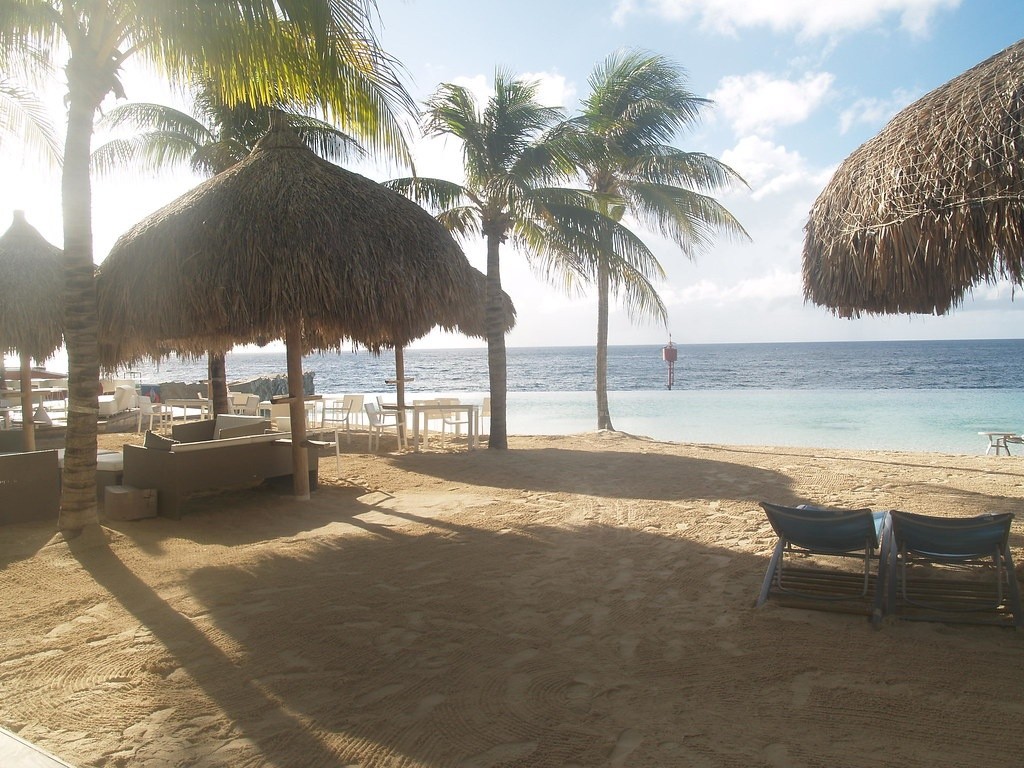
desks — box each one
[165,399,208,424]
[383,406,478,453]
[260,400,316,428]
[978,432,1016,456]
[54,449,123,502]
[0,378,68,426]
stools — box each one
[105,485,157,520]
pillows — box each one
[144,429,180,452]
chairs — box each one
[757,502,1024,631]
[98,378,490,473]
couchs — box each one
[123,414,318,520]
